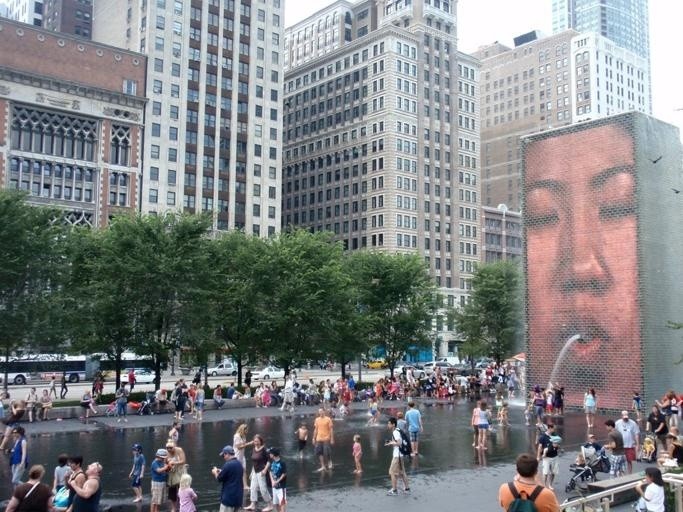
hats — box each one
[131,443,143,451]
[266,446,281,455]
[534,384,540,390]
[219,446,235,456]
[587,433,597,440]
[155,448,168,459]
[621,410,630,419]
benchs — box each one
[588,466,668,508]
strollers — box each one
[565,444,611,495]
[637,432,658,462]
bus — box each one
[0,353,156,385]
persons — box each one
[523,123,641,410]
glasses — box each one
[166,447,173,449]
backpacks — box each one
[507,482,544,512]
[392,429,412,455]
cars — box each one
[206,361,238,378]
[250,364,285,380]
[474,358,490,368]
[490,360,506,368]
[384,362,453,379]
[366,360,387,369]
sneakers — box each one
[386,488,399,496]
[400,488,411,495]
